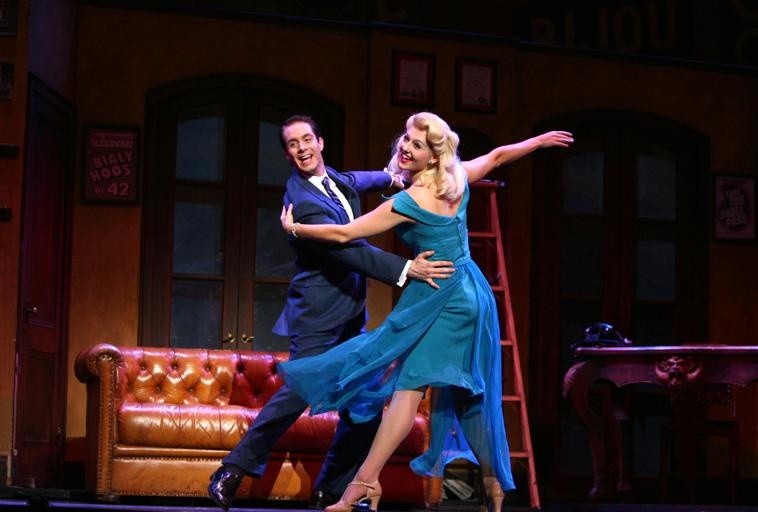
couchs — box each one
[72,342,445,510]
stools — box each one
[666,418,740,506]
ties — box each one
[321,176,347,222]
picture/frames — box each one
[388,47,438,111]
[452,54,499,116]
[709,166,758,246]
[79,120,142,209]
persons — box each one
[280,112,575,512]
[564,323,703,501]
[207,113,455,511]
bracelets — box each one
[291,222,301,239]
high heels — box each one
[482,479,505,512]
[324,478,383,512]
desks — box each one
[562,339,758,512]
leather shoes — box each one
[208,464,243,511]
[313,488,370,512]
